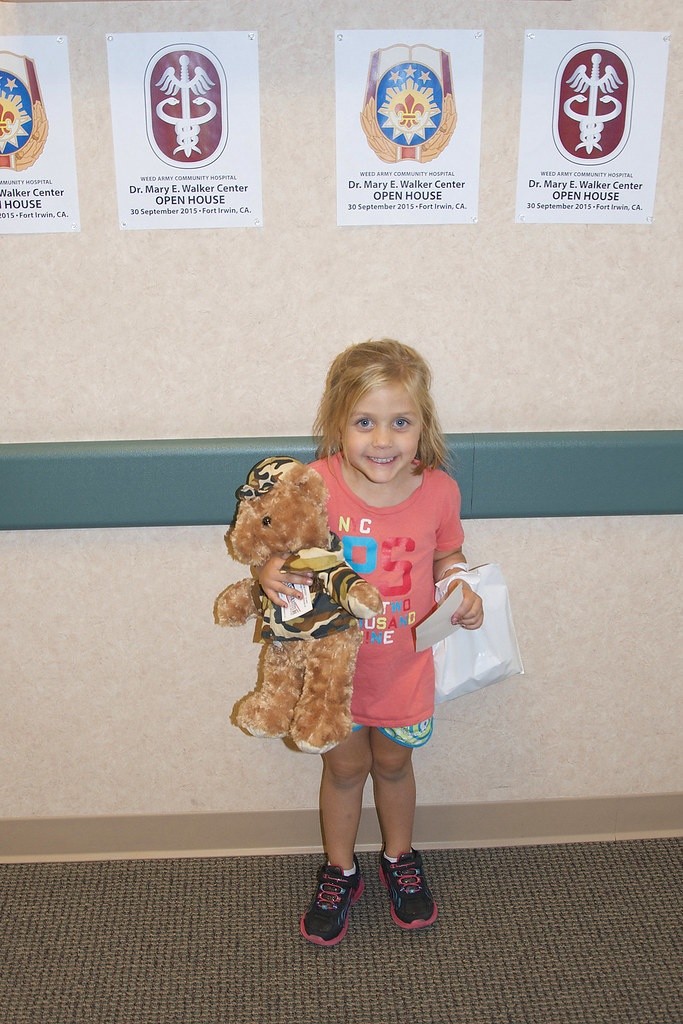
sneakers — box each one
[299,853,363,946]
[377,846,438,930]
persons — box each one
[295,340,485,947]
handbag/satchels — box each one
[432,561,524,706]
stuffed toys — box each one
[210,453,389,757]
